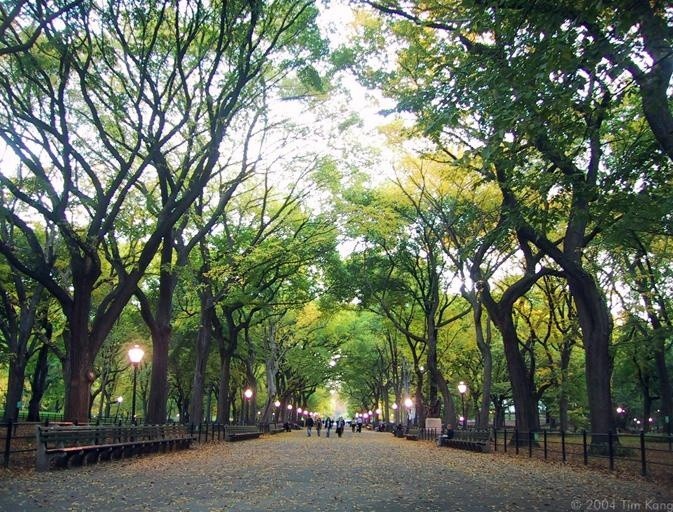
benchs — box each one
[397,422,492,450]
[35,422,298,471]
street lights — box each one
[457,379,469,430]
[125,342,145,423]
[356,396,412,432]
[113,395,125,427]
[614,405,661,435]
[241,389,321,432]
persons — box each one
[438,424,454,446]
[283,422,291,432]
[350,417,362,433]
[305,415,345,437]
[394,424,403,437]
